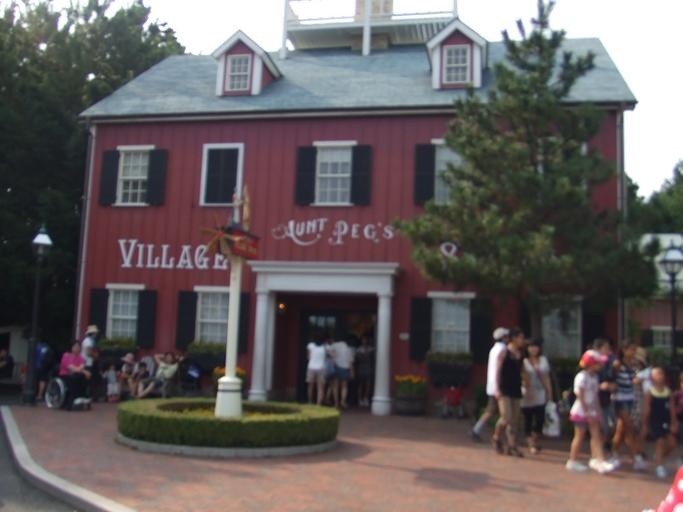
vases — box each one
[395,398,427,415]
[214,387,245,400]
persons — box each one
[306,332,375,408]
[469,328,683,479]
[0,348,14,381]
[35,325,209,415]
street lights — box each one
[19,223,53,407]
[657,243,683,389]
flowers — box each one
[212,367,247,387]
[393,374,428,398]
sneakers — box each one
[469,429,541,458]
[566,451,667,479]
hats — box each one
[579,350,609,368]
[84,324,99,334]
[122,353,134,364]
[494,328,510,339]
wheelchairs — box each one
[43,368,94,410]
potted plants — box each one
[98,337,140,371]
[423,352,470,388]
[185,338,226,376]
